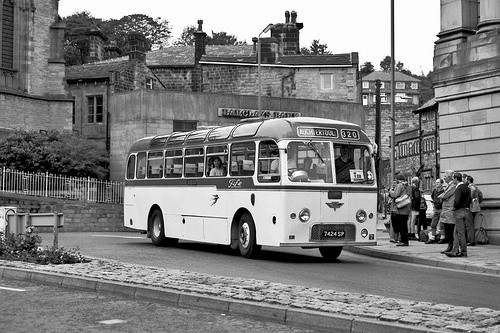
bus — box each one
[123,117,378,260]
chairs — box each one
[140,158,269,178]
[313,163,327,177]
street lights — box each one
[257,24,275,116]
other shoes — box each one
[467,242,475,246]
[396,242,409,246]
[445,251,458,257]
[458,252,467,257]
[441,245,452,254]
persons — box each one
[271,152,297,174]
[428,179,445,244]
[416,189,428,241]
[301,158,315,179]
[407,177,421,241]
[445,172,469,257]
[209,157,226,177]
[335,146,355,183]
[390,173,412,246]
[461,174,482,246]
[437,170,458,255]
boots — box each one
[425,236,435,244]
[408,233,419,240]
[438,234,446,244]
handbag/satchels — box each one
[475,227,489,245]
[395,195,412,208]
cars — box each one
[412,195,434,225]
